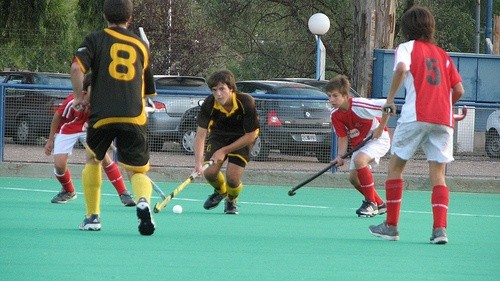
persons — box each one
[194,70,259,214]
[324,74,391,217]
[44,74,136,206]
[368,6,464,244]
[70,0,155,235]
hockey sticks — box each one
[288,134,373,196]
[384,104,467,121]
[154,157,214,213]
[75,98,155,113]
[110,144,166,207]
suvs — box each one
[144,74,213,150]
[0,70,74,145]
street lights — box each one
[308,13,331,80]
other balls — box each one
[173,205,182,213]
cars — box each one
[267,78,361,112]
[178,80,338,164]
[484,110,500,158]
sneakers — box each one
[356,200,378,218]
[79,215,101,230]
[368,223,399,241]
[430,227,448,244]
[224,198,238,214]
[137,197,155,236]
[51,191,77,203]
[120,192,136,206]
[378,202,387,214]
[204,189,227,210]
